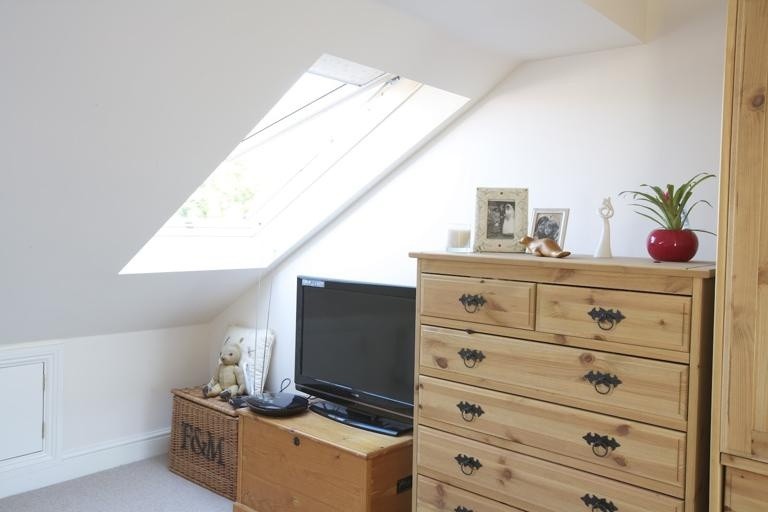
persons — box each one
[502,203,514,235]
[489,208,500,236]
[544,220,560,243]
[533,216,549,239]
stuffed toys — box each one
[202,341,246,399]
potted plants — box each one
[618,172,717,261]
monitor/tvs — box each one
[294,275,415,437]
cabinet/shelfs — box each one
[232,406,412,512]
[406,248,716,512]
[706,0,768,512]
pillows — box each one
[219,325,276,395]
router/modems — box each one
[243,392,308,418]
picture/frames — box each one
[472,187,569,255]
